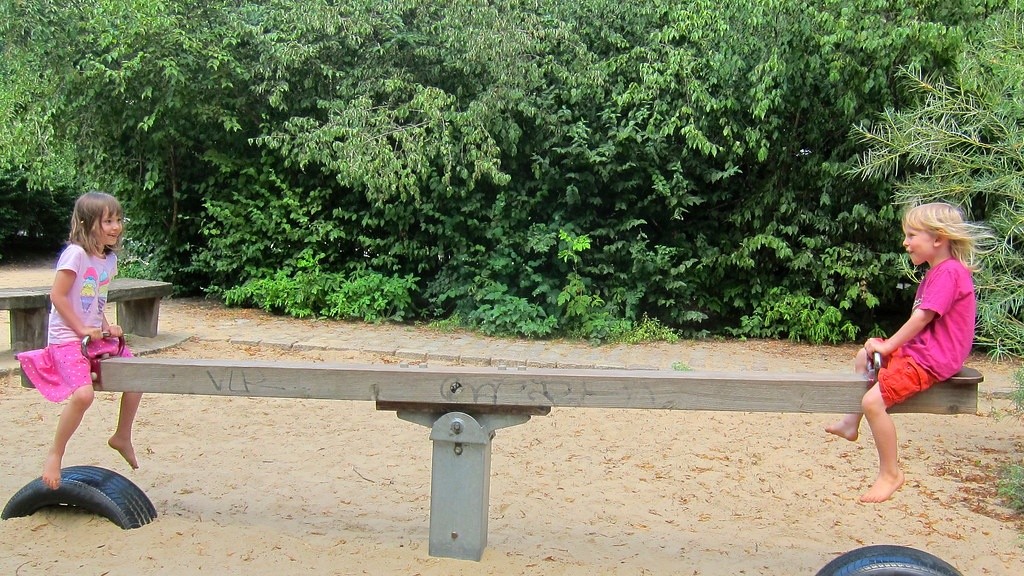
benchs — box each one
[0,279,173,352]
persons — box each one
[825,205,996,501]
[16,194,142,487]
